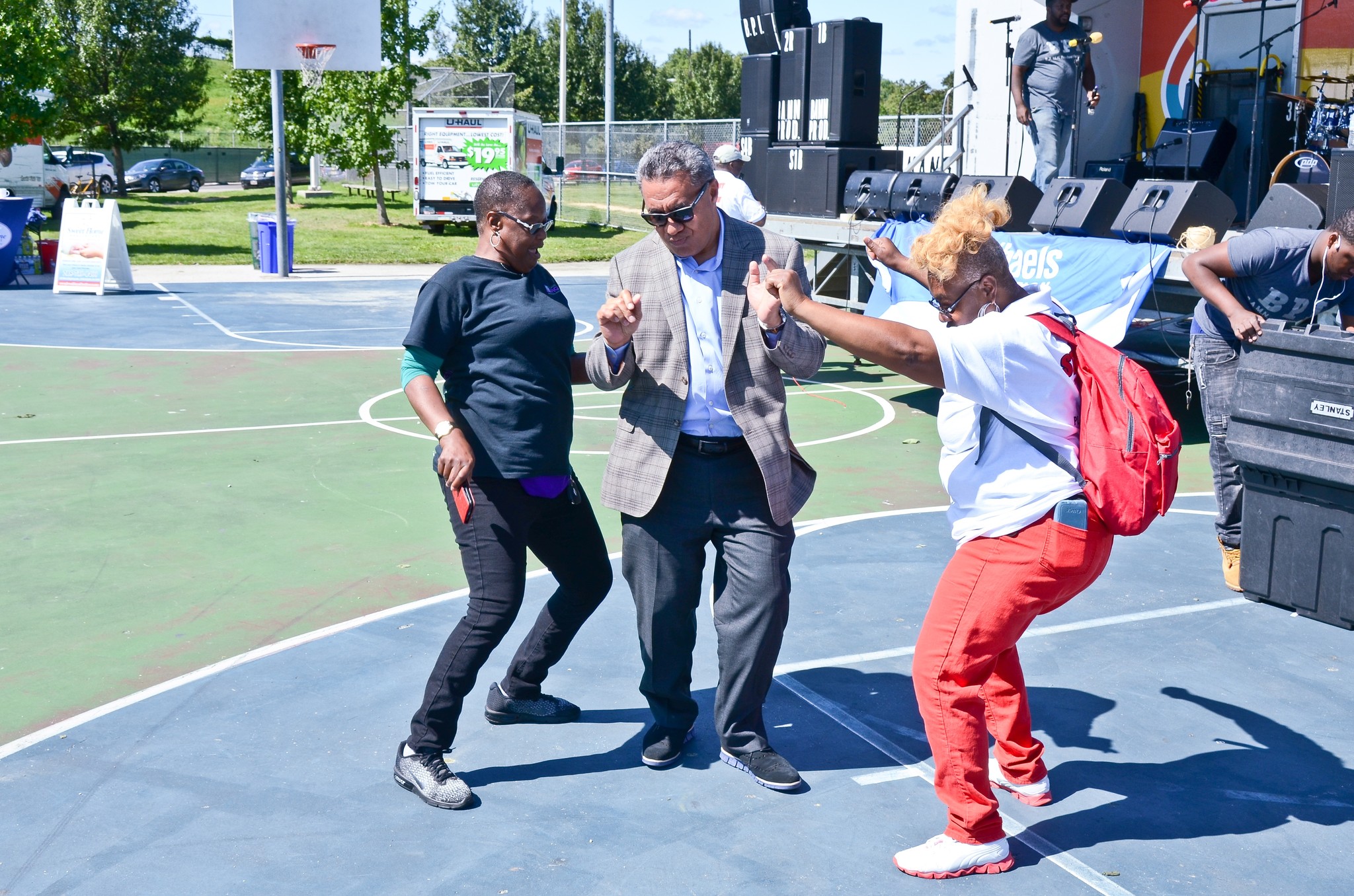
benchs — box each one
[341,183,401,203]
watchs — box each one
[754,308,786,334]
[435,421,459,441]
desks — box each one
[25,207,47,276]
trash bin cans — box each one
[248,212,290,270]
[259,219,295,273]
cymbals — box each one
[1268,91,1315,107]
[1296,72,1354,84]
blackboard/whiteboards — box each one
[54,197,133,291]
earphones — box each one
[1335,235,1341,248]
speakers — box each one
[1110,179,1238,250]
[739,134,777,205]
[740,51,778,134]
[808,20,883,147]
[738,0,811,54]
[1028,177,1132,241]
[1084,159,1150,190]
[1242,181,1329,235]
[892,172,959,221]
[1236,97,1318,228]
[765,146,902,219]
[1325,148,1353,230]
[1142,117,1237,180]
[778,26,811,144]
[842,170,904,221]
[945,175,1046,232]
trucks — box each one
[411,106,557,234]
[420,143,468,168]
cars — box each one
[113,158,206,193]
[239,152,307,190]
[564,159,603,181]
[602,160,639,179]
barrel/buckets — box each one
[34,240,58,273]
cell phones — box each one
[452,477,476,524]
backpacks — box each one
[979,312,1186,536]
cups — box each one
[0,188,10,198]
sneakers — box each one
[485,683,579,724]
[640,722,696,768]
[393,738,472,809]
[987,756,1052,810]
[1221,544,1245,594]
[720,745,801,789]
[893,832,1014,879]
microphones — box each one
[1087,85,1098,115]
[1157,138,1182,150]
[1183,0,1209,8]
[991,15,1021,24]
[1069,32,1102,47]
[963,64,977,91]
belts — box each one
[678,431,744,457]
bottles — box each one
[50,259,55,274]
[14,232,41,275]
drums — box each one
[1304,102,1348,148]
[1269,150,1330,190]
[1337,103,1354,138]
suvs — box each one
[44,150,116,195]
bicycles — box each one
[69,172,103,200]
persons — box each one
[773,181,1113,881]
[1181,211,1354,594]
[393,173,613,810]
[586,140,829,790]
[1011,0,1101,196]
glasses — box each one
[641,178,713,226]
[930,278,982,317]
[499,211,558,238]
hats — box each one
[713,145,751,164]
